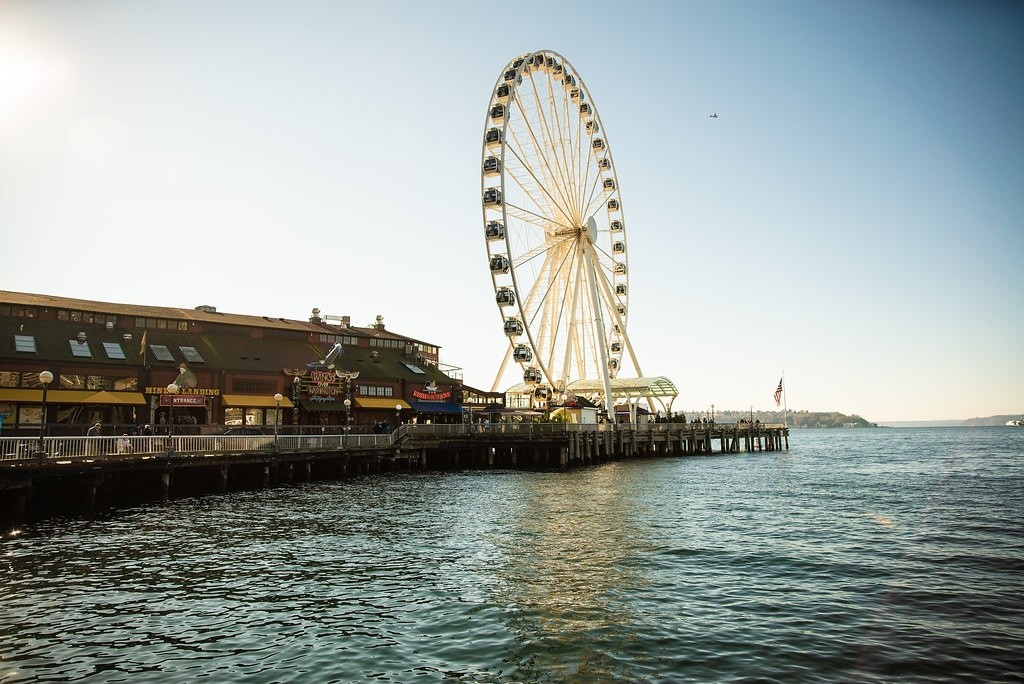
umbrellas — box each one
[501,411,543,416]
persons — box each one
[373,419,390,434]
[144,426,152,436]
[86,423,101,455]
[774,378,783,407]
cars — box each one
[224,427,265,435]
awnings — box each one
[0,389,146,404]
[410,401,469,413]
[300,401,347,410]
[355,398,411,408]
[221,395,294,407]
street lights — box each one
[396,403,402,446]
[273,393,283,454]
[666,403,670,430]
[467,396,473,423]
[36,370,54,465]
[344,399,352,449]
[711,404,714,432]
[750,405,754,423]
[562,395,567,420]
[167,383,179,457]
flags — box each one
[140,331,146,354]
